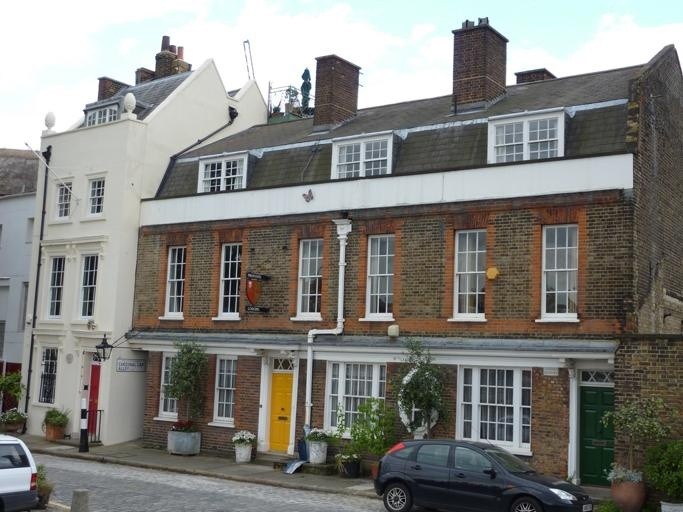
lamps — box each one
[95,332,138,362]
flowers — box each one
[331,402,361,460]
[0,409,28,423]
[232,429,256,445]
[306,427,333,442]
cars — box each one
[372,436,595,512]
[496,416,529,439]
[0,434,40,512]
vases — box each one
[234,445,252,466]
[0,423,24,435]
[308,444,329,466]
[337,457,360,480]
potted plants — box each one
[352,398,397,482]
[42,407,71,443]
[35,465,52,504]
[600,392,668,512]
[642,438,683,512]
[163,332,206,455]
